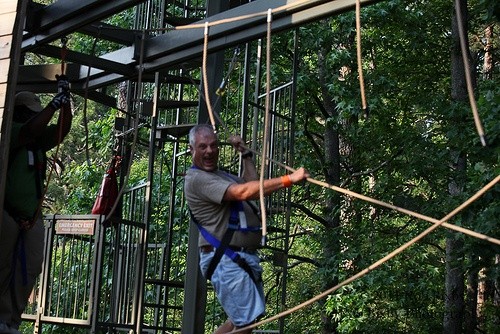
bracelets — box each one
[281,175,292,188]
[241,151,253,160]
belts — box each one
[199,244,258,255]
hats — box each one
[13,91,44,113]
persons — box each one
[183,124,311,334]
[0,74,74,334]
